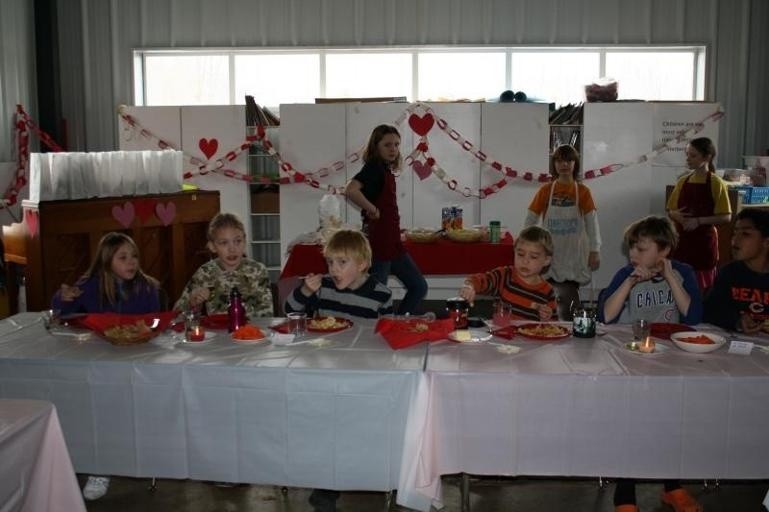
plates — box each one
[641,321,697,341]
[171,330,219,344]
[93,324,160,345]
[619,339,671,356]
[229,327,267,343]
[302,315,353,333]
[446,329,492,343]
[516,323,569,341]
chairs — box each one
[442,293,506,321]
[590,286,723,496]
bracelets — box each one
[373,207,377,216]
[696,217,700,225]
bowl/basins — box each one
[403,228,440,243]
[668,331,726,353]
[447,229,483,242]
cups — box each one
[446,308,469,330]
[42,309,60,332]
[630,318,653,339]
[492,302,513,329]
[155,311,179,348]
[181,309,200,340]
[286,312,306,342]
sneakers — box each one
[83,474,111,500]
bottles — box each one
[227,286,247,334]
[318,191,341,232]
[487,220,501,243]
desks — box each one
[663,181,768,288]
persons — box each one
[50,232,160,501]
[596,216,706,512]
[171,214,274,488]
[281,230,394,512]
[665,138,732,297]
[523,145,601,321]
[459,225,559,320]
[703,209,769,334]
[344,125,427,314]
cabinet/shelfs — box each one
[280,103,413,269]
[119,105,252,256]
[413,102,549,233]
[579,103,718,303]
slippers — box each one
[661,486,703,511]
[615,504,640,512]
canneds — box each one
[490,221,500,243]
[447,298,470,329]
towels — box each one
[202,314,250,330]
[644,319,700,340]
